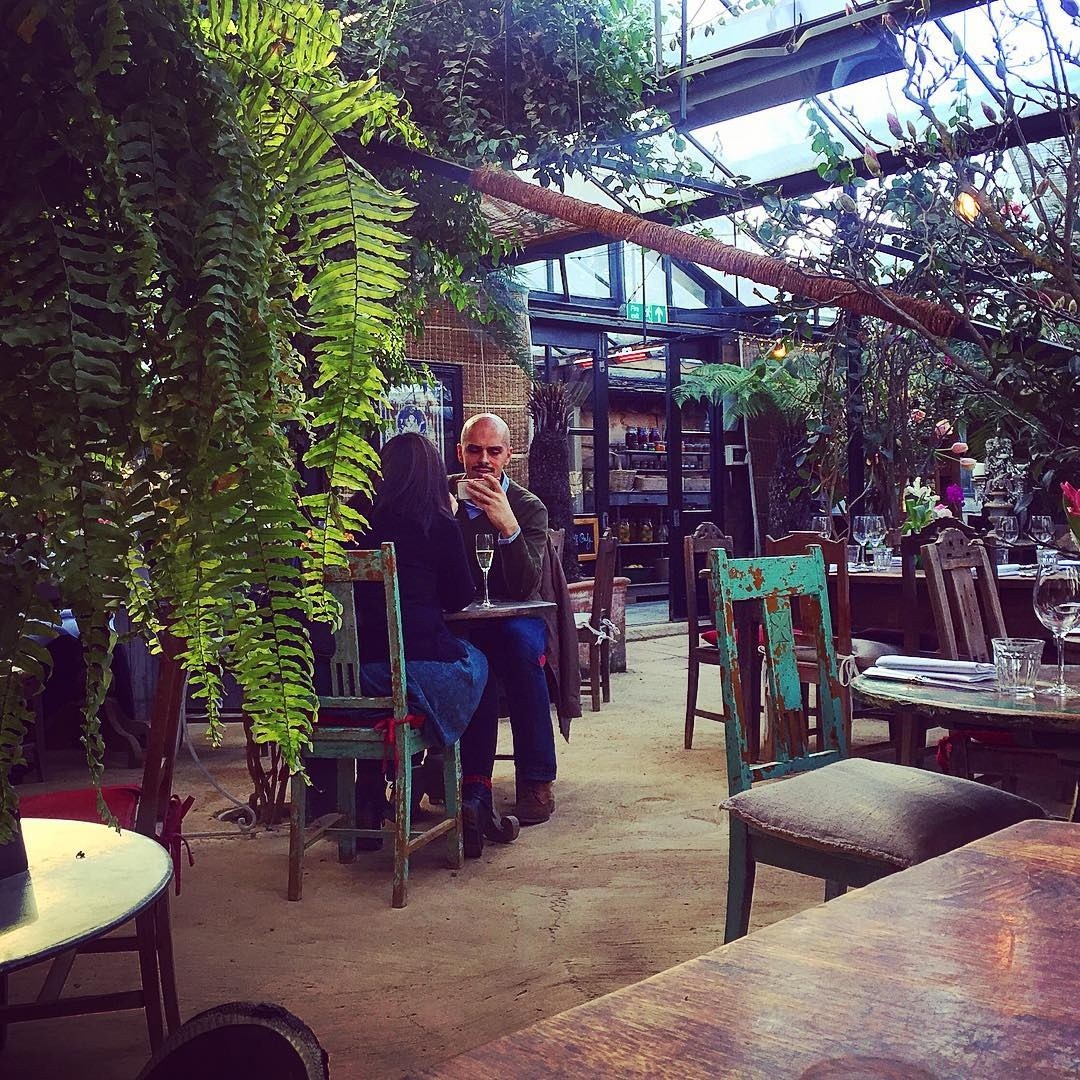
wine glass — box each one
[1033,563,1080,698]
[1028,516,1060,569]
[476,534,497,607]
[995,514,1020,572]
[853,516,887,572]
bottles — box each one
[611,517,670,544]
[626,426,712,468]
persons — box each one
[318,434,499,859]
[445,413,557,823]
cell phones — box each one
[456,479,493,499]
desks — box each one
[411,815,1080,1079]
[444,595,560,841]
[846,661,1079,825]
[818,560,1079,662]
[0,817,183,1080]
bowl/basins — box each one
[623,567,654,583]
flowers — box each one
[900,482,951,566]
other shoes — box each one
[355,800,383,851]
[460,798,489,860]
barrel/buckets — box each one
[656,557,670,582]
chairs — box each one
[280,541,463,907]
[1,582,195,1058]
[557,525,1080,791]
[703,542,1038,952]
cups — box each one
[811,517,861,572]
[992,638,1046,694]
[872,548,892,573]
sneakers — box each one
[513,775,554,824]
[387,782,425,817]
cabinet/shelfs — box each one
[612,428,712,548]
